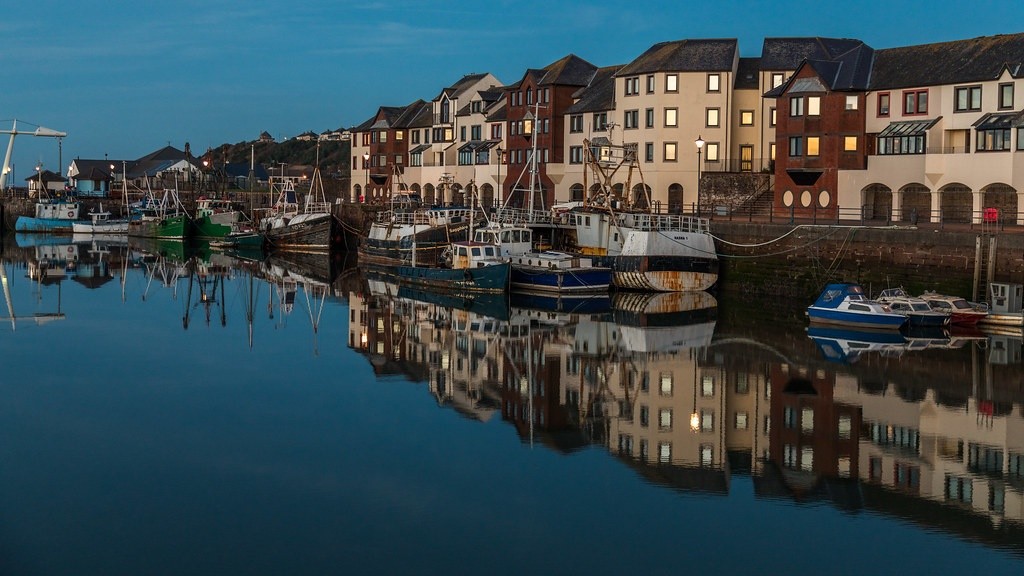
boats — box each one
[15,95,722,449]
[803,321,1024,367]
[876,276,953,328]
[805,283,910,331]
[966,281,1024,328]
[917,290,990,327]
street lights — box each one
[203,160,208,198]
[363,152,371,197]
[695,135,706,217]
[495,145,503,209]
[109,164,115,200]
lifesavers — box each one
[68,246,73,252]
[464,271,472,280]
[463,300,472,310]
[198,217,204,224]
[480,221,486,227]
[68,212,74,217]
[266,223,272,231]
[162,220,167,226]
[160,251,167,257]
